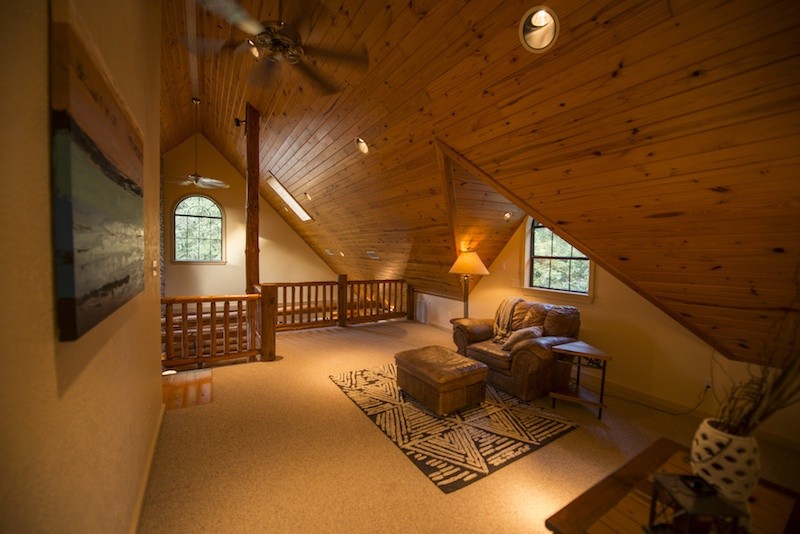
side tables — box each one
[549,340,613,420]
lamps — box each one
[448,251,490,319]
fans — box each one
[167,0,369,96]
[165,97,231,190]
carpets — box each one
[329,362,582,494]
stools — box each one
[394,336,489,417]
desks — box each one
[546,436,800,534]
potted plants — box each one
[690,287,800,502]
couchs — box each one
[501,326,544,350]
[450,297,581,402]
